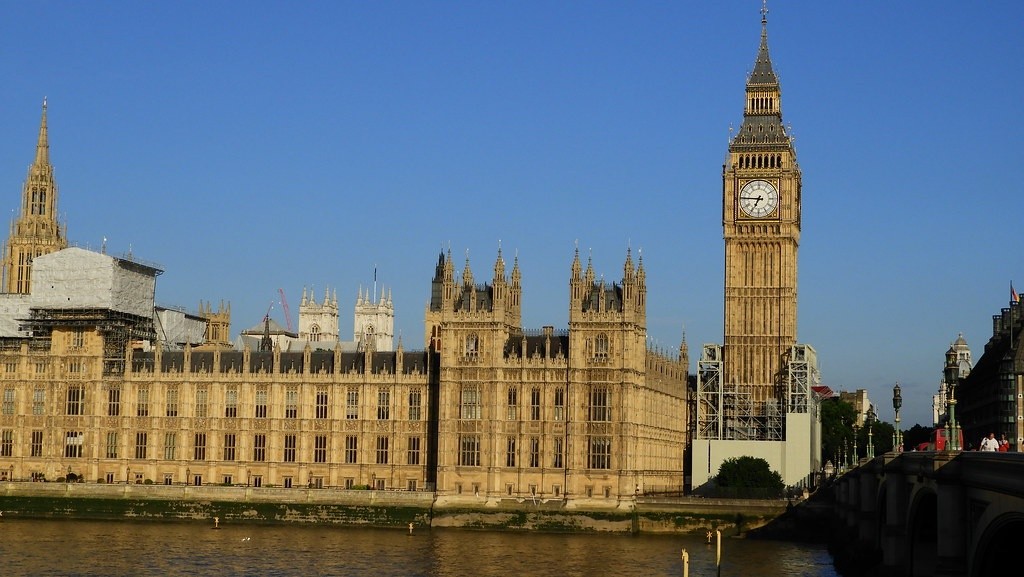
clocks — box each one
[740,179,778,218]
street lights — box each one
[837,445,841,474]
[834,454,837,475]
[843,437,848,470]
[944,360,960,452]
[852,424,859,470]
[893,382,903,454]
[867,408,873,460]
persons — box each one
[979,433,999,452]
[999,434,1010,452]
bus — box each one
[929,429,963,451]
[911,443,931,451]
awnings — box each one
[812,386,833,403]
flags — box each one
[1012,288,1019,302]
